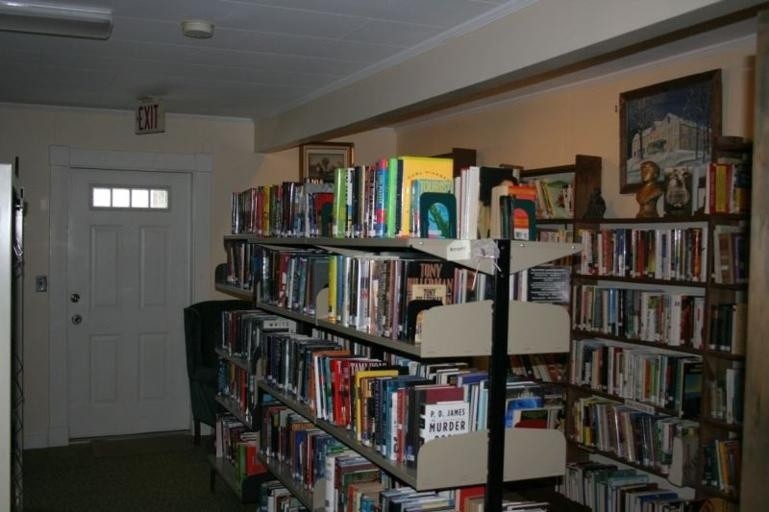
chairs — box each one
[183,295,259,447]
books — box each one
[576,221,748,511]
[213,157,575,511]
[663,158,747,217]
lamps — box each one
[1,0,115,43]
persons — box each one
[636,161,663,218]
[583,187,606,219]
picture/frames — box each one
[614,65,724,195]
[296,139,356,187]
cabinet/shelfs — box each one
[0,154,36,512]
[206,185,585,510]
[519,149,750,511]
